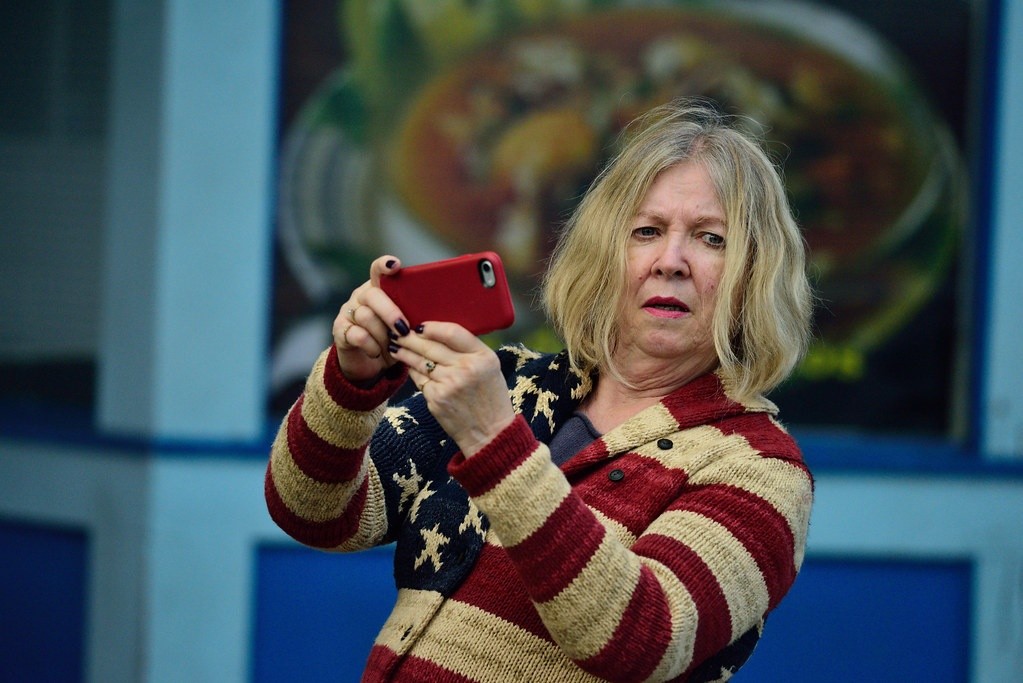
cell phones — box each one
[380,252,516,337]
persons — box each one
[265,97,814,683]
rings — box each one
[346,304,365,323]
[417,378,431,392]
[342,323,352,344]
[426,361,435,378]
